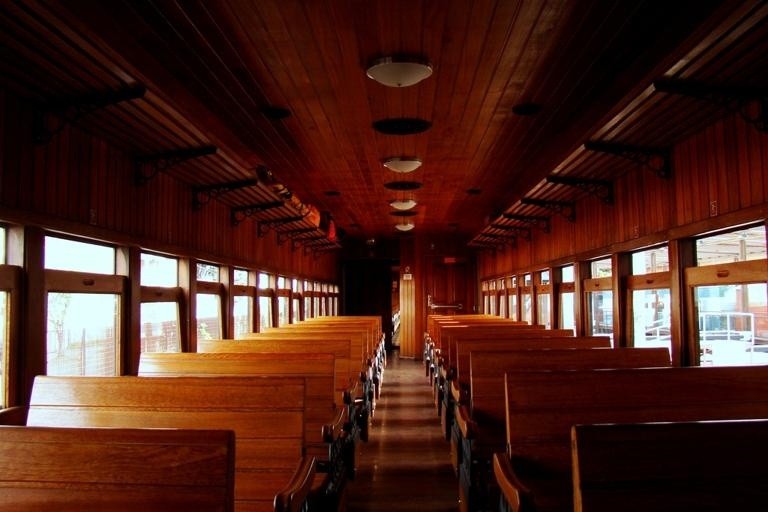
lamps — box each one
[397,223,416,231]
[382,156,425,177]
[388,198,418,212]
[366,50,435,91]
